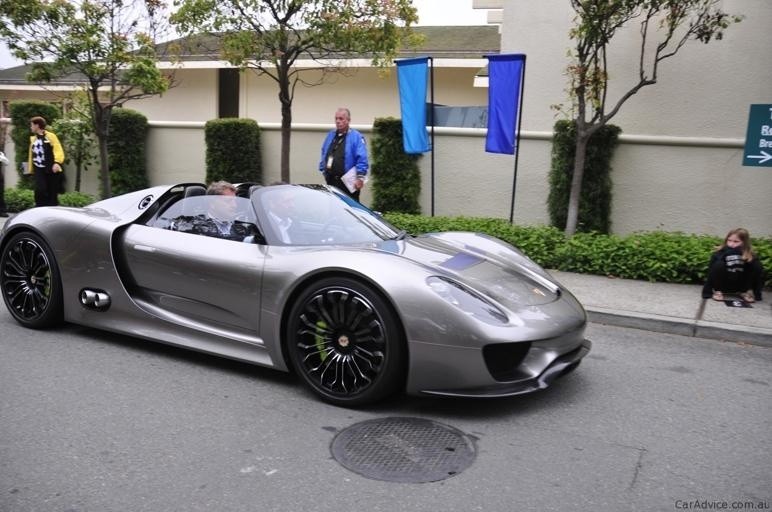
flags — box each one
[485,54,523,155]
[396,56,433,154]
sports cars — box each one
[0,180,591,409]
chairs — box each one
[184,185,207,197]
[249,185,263,197]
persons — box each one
[701,228,765,303]
[0,144,8,217]
[28,117,65,207]
[319,108,370,204]
[188,181,313,246]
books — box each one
[341,166,369,194]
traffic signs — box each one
[740,104,771,166]
